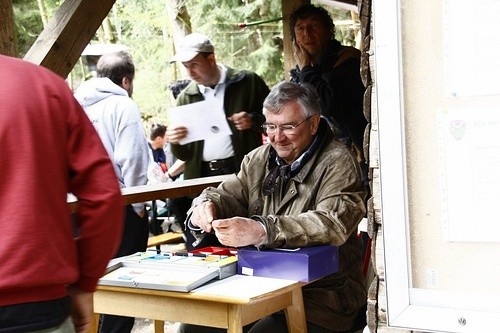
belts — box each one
[197,159,231,171]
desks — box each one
[89,250,329,333]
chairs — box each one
[355,217,372,278]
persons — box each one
[145,122,169,176]
[168,77,191,100]
[0,51,126,332]
[162,129,177,168]
[158,31,273,252]
[284,3,371,216]
[71,50,150,332]
[182,80,370,332]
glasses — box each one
[261,113,317,134]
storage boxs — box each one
[96,245,237,293]
[237,242,341,283]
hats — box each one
[169,33,215,63]
